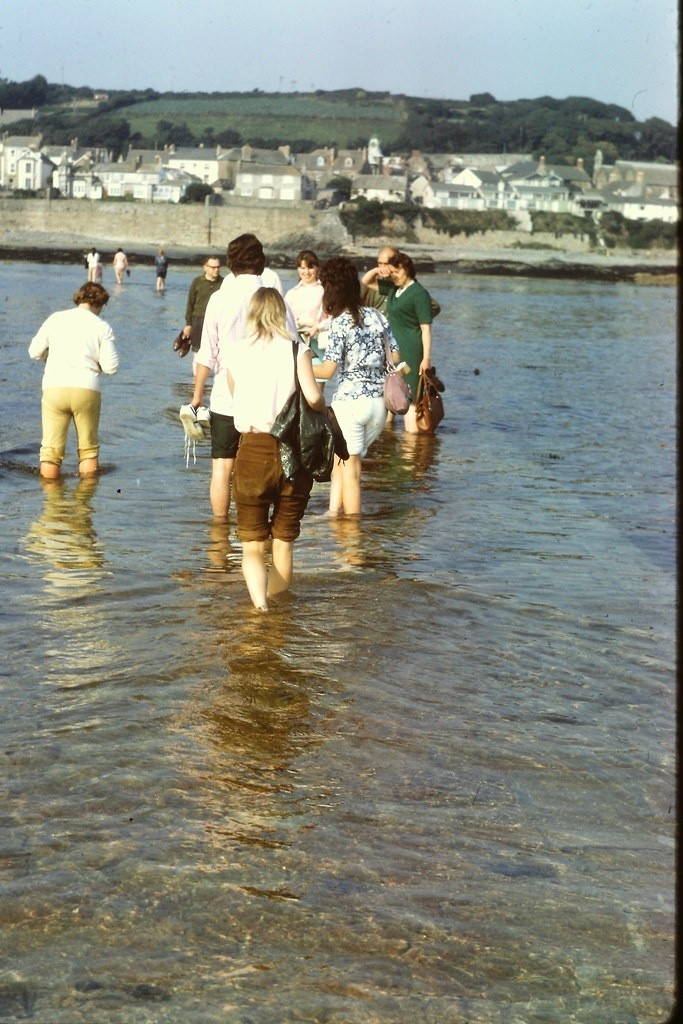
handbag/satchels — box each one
[415,374,444,436]
[371,308,413,415]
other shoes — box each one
[173,330,191,358]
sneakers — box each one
[180,404,205,469]
[196,406,211,428]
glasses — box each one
[206,265,220,270]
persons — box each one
[183,232,299,517]
[359,245,441,433]
[226,288,325,611]
[311,255,399,516]
[283,246,334,364]
[28,282,119,478]
[113,248,129,284]
[154,248,168,291]
[87,248,99,282]
